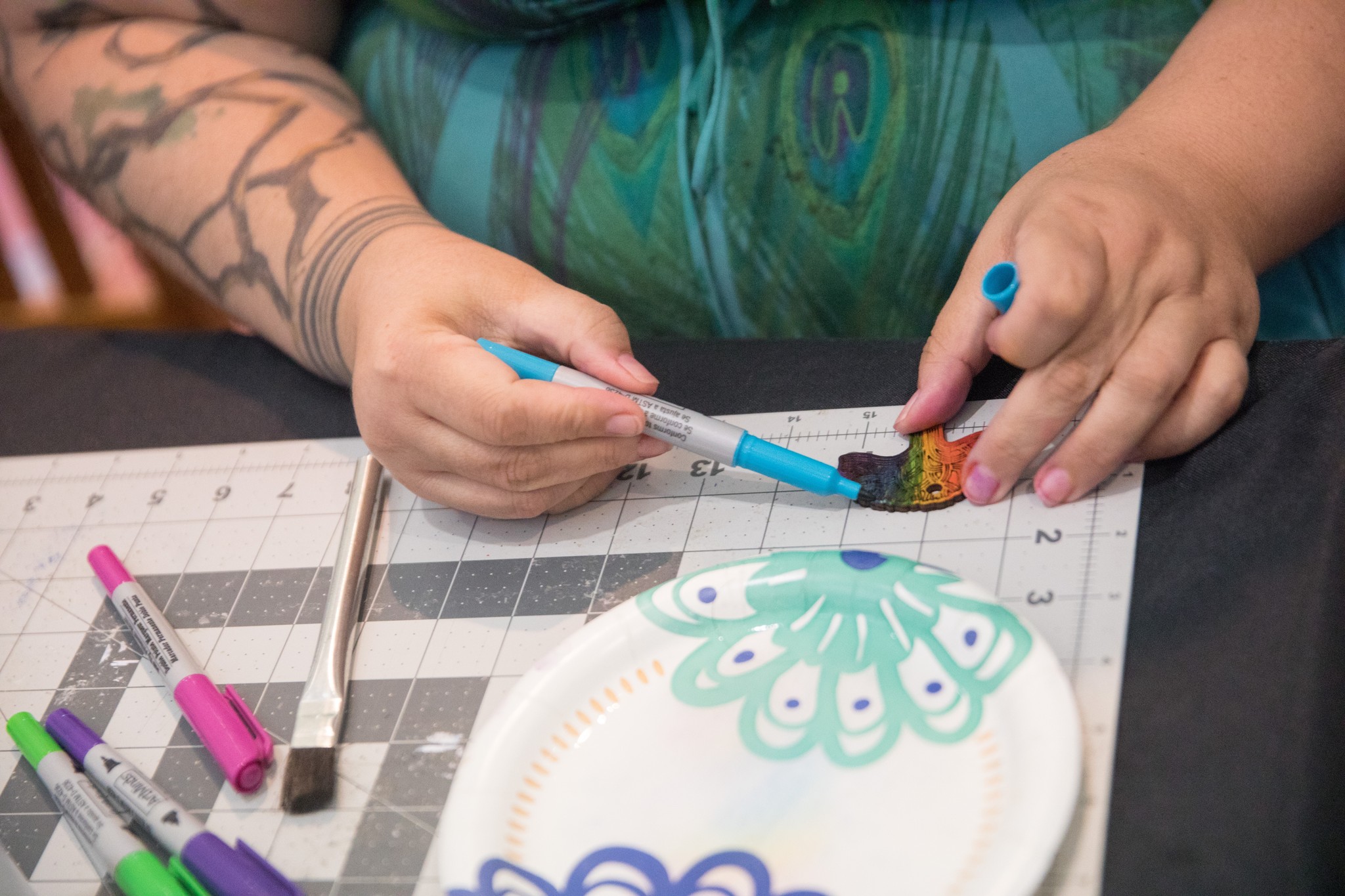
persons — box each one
[0,0,1345,521]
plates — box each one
[438,547,1085,896]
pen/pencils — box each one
[88,543,272,794]
[475,338,876,499]
[45,708,302,896]
[6,712,208,895]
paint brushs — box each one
[278,451,381,812]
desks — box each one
[0,314,1345,896]
